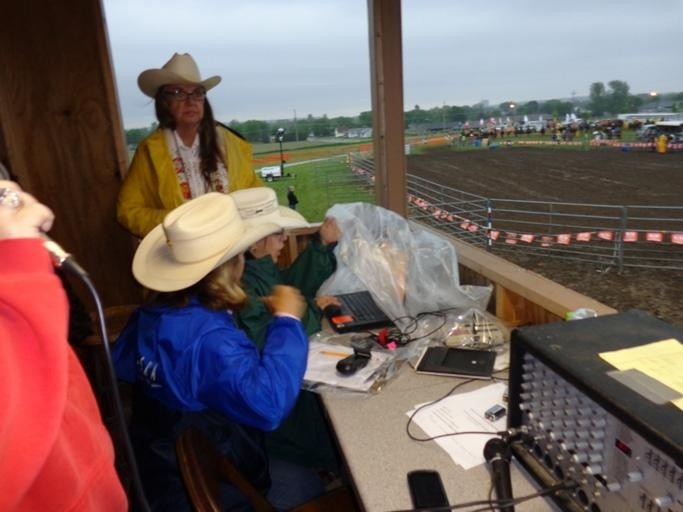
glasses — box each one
[161,88,207,102]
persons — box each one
[115,79,266,242]
[450,113,664,146]
[286,185,299,210]
[223,214,344,350]
[109,248,327,510]
[0,173,130,511]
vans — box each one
[634,124,656,139]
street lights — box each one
[277,127,286,177]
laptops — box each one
[323,239,411,334]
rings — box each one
[7,192,23,210]
[0,189,7,202]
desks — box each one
[308,297,569,511]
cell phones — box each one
[407,470,452,512]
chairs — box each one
[175,424,362,512]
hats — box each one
[131,190,281,293]
[230,187,312,234]
[137,52,222,98]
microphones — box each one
[483,438,515,512]
[0,187,87,280]
